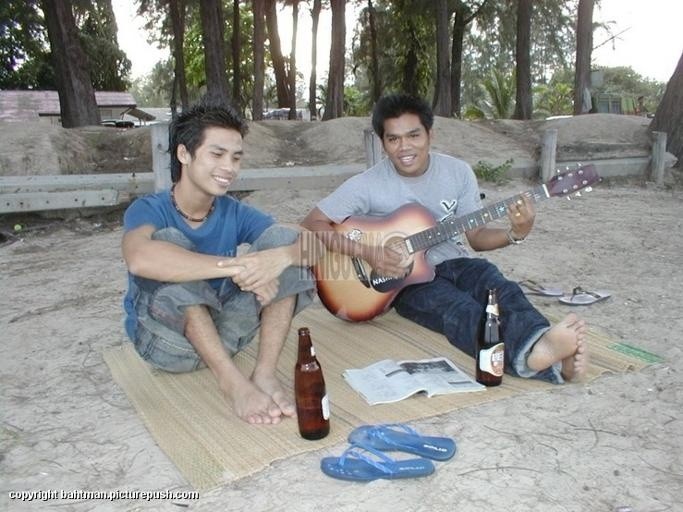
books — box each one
[340,356,487,406]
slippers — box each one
[559,284,611,307]
[514,279,563,298]
[346,422,456,462]
[319,444,435,482]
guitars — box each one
[310,163,602,323]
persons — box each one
[636,95,648,114]
[299,93,586,386]
[121,102,318,425]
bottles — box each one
[475,286,505,387]
[293,327,330,440]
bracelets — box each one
[505,229,527,245]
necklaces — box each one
[169,181,216,223]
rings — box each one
[514,212,520,217]
